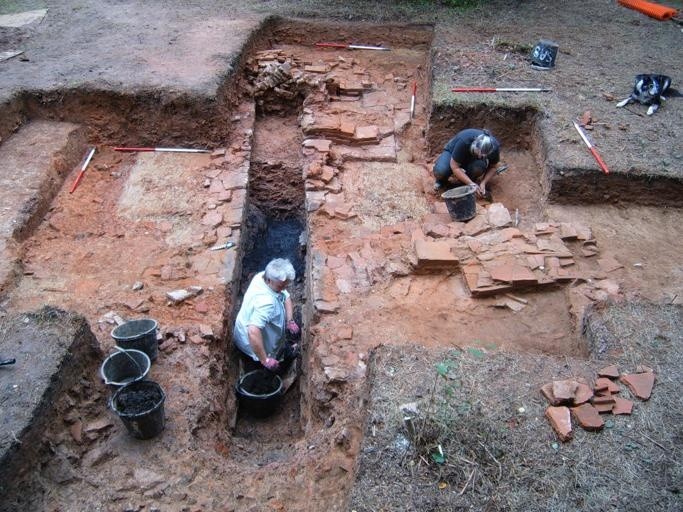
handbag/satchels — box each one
[533,39,557,67]
[631,73,669,104]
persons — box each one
[433,126,501,202]
[233,255,303,374]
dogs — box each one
[616,73,683,116]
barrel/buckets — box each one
[235,370,282,420]
[110,378,166,440]
[441,184,477,221]
[102,345,151,392]
[532,39,559,71]
[111,318,158,361]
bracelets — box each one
[287,319,294,323]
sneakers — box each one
[434,178,445,191]
